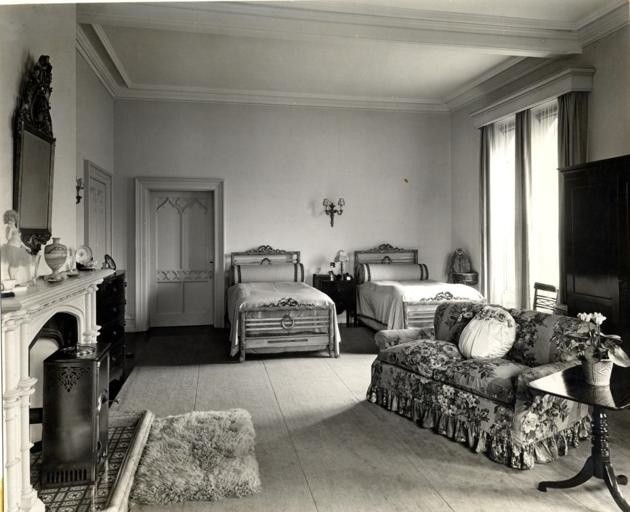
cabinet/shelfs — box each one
[96,269,128,382]
[558,155,629,360]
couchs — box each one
[366,302,601,472]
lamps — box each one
[75,177,86,206]
[334,250,350,281]
[323,197,345,227]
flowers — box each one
[568,311,630,368]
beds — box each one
[225,244,342,363]
[352,243,486,332]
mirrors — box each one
[10,55,57,254]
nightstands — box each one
[312,274,357,328]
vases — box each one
[44,235,69,283]
[580,357,613,387]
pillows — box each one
[356,262,429,284]
[457,305,518,361]
[232,263,305,284]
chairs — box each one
[532,282,559,312]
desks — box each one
[527,364,630,512]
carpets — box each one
[127,408,262,504]
